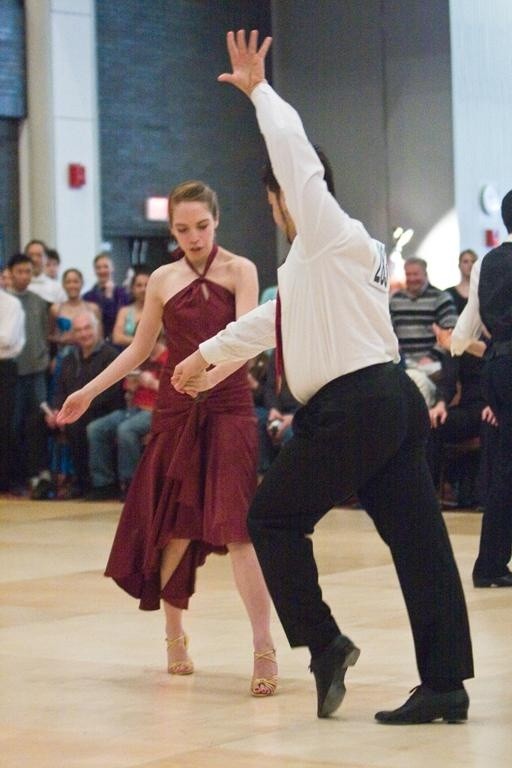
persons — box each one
[169,29,474,725]
[242,286,304,474]
[0,236,166,505]
[388,245,500,509]
[59,183,277,698]
[432,189,512,590]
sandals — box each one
[165,633,194,676]
[250,650,278,698]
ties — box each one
[275,290,283,397]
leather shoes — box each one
[9,478,125,500]
[375,684,470,723]
[309,635,361,717]
[473,567,512,587]
